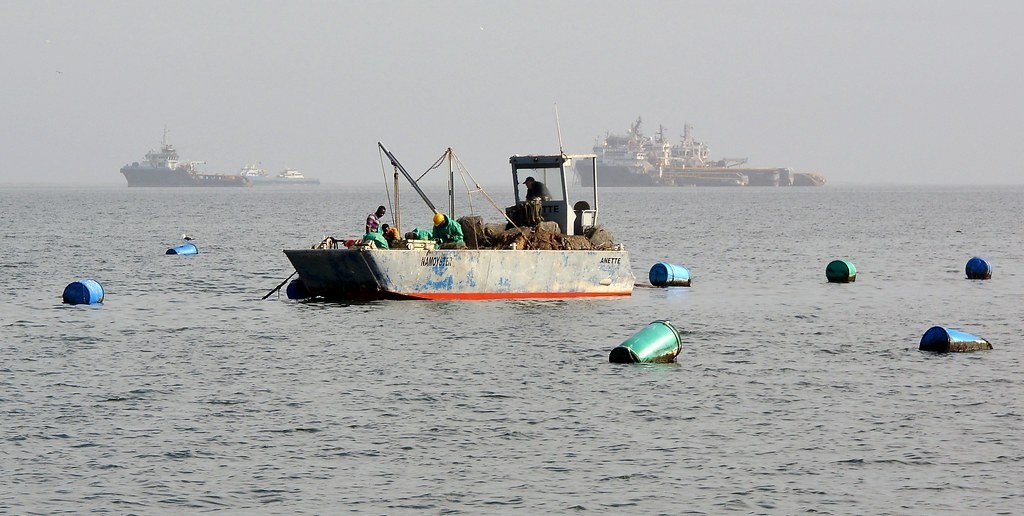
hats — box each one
[523,177,534,184]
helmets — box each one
[433,213,444,226]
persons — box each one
[381,223,403,241]
[525,176,552,202]
[432,212,464,249]
[366,205,386,233]
[405,227,434,241]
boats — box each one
[284,102,638,300]
[118,124,254,188]
[239,163,320,185]
[575,119,826,189]
